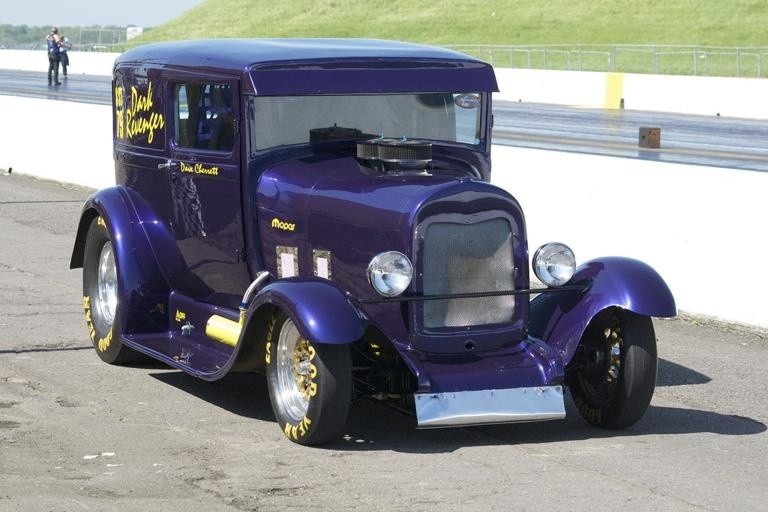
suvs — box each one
[70,37,678,444]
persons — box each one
[52,35,72,79]
[45,27,61,86]
